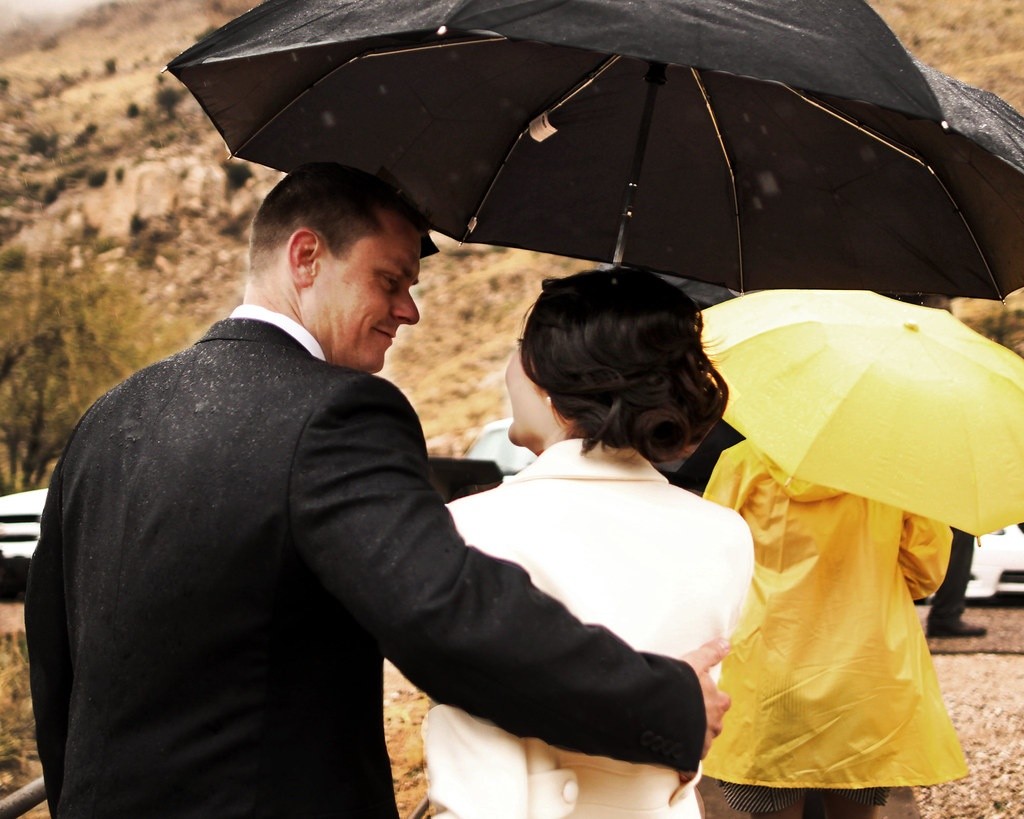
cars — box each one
[972,525,1024,594]
[464,421,536,475]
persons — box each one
[421,267,753,819]
[925,527,986,638]
[699,439,968,819]
[24,164,732,819]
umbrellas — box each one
[695,289,1024,548]
[157,0,1024,309]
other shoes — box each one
[927,617,987,638]
